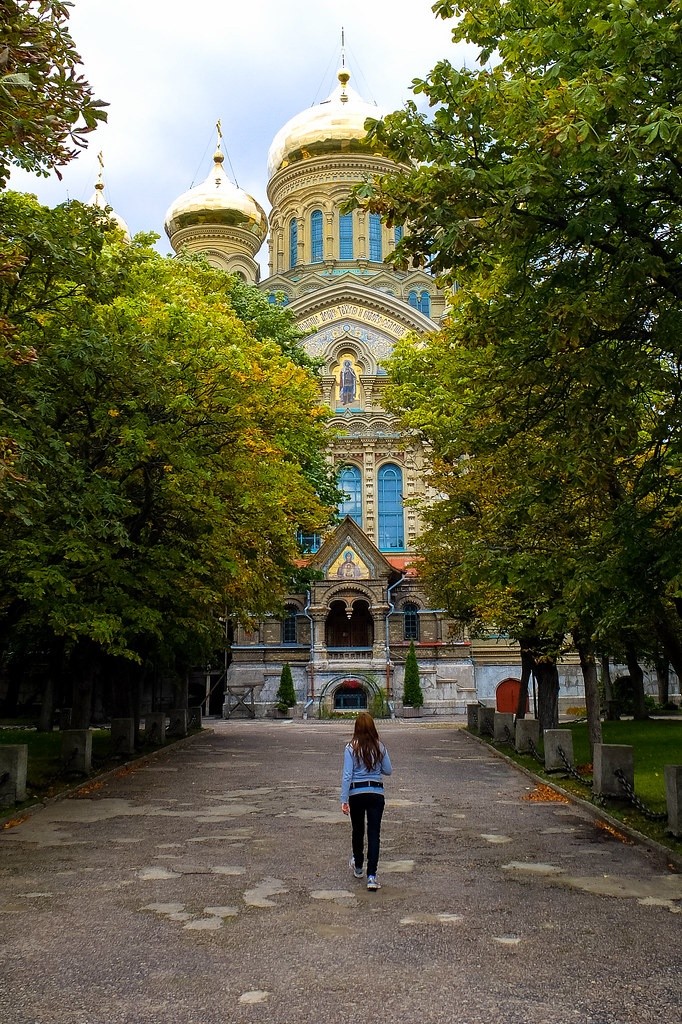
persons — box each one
[341,712,392,888]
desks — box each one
[223,684,264,719]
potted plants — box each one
[402,638,424,717]
[272,661,297,719]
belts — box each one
[349,781,384,790]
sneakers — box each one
[367,878,382,888]
[349,857,363,878]
